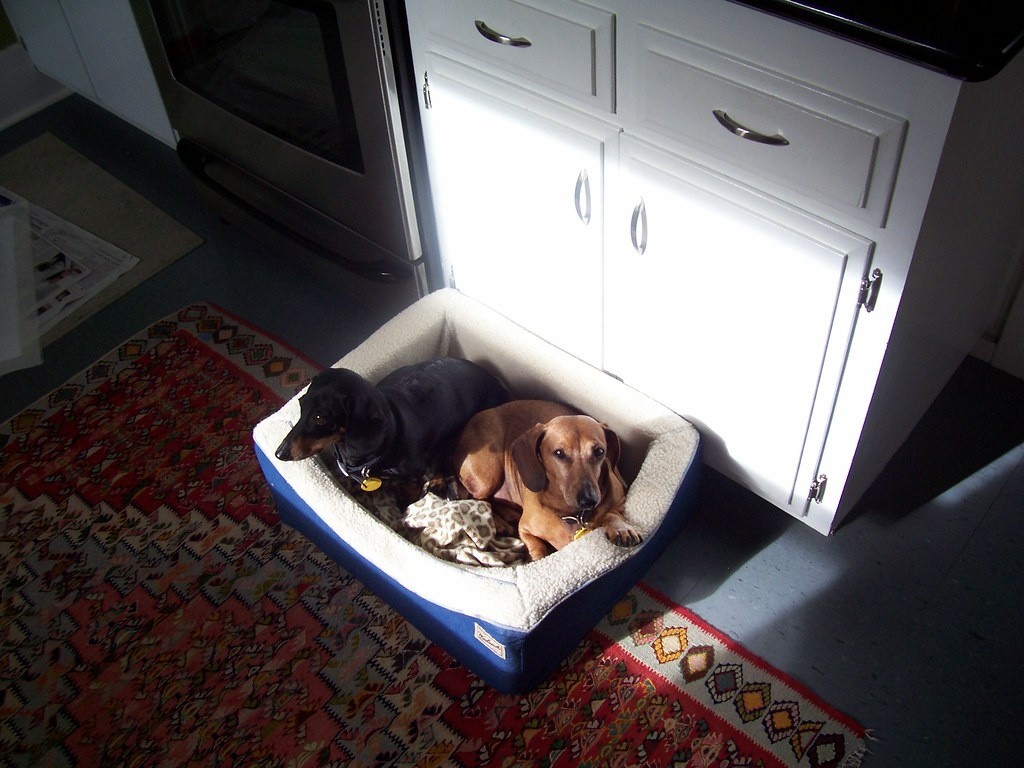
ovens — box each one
[130,0,443,312]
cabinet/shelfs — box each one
[401,0,1023,539]
[0,1,180,151]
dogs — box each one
[275,357,644,563]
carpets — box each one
[1,302,883,768]
[0,130,204,351]
